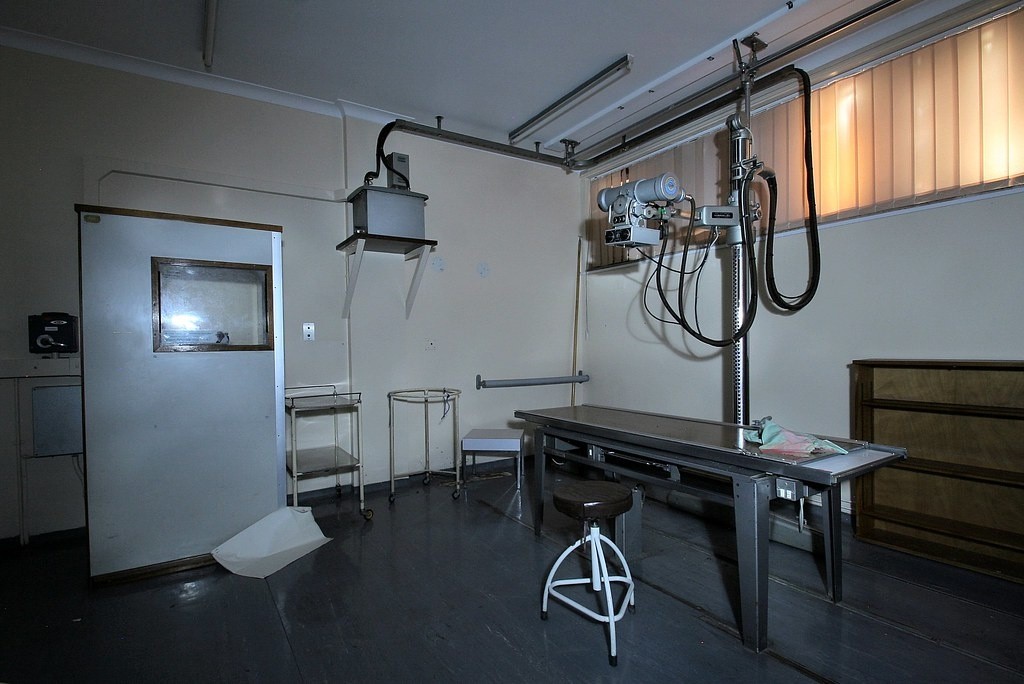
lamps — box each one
[508,53,636,145]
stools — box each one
[540,480,636,668]
